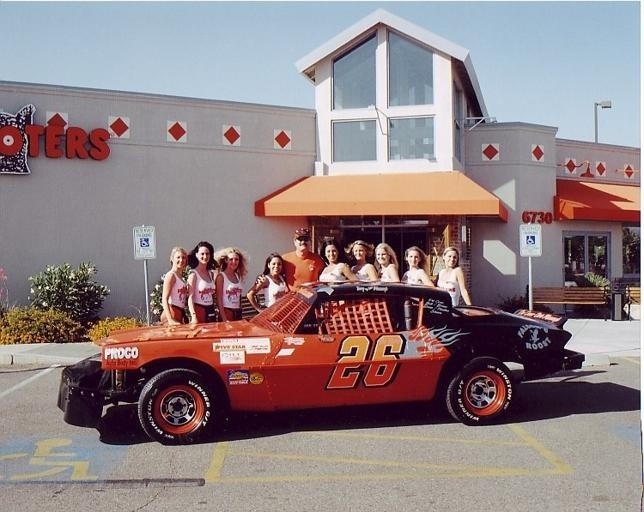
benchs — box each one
[526,286,641,320]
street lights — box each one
[594,101,612,144]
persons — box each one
[282,226,325,291]
[213,247,248,322]
[400,246,434,286]
[344,240,380,282]
[187,240,219,324]
[434,247,473,306]
[158,246,189,325]
[319,240,359,283]
[247,252,290,314]
[374,243,400,283]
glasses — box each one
[296,236,309,241]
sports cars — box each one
[56,280,586,447]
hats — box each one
[295,225,312,239]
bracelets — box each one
[256,303,262,311]
[190,312,196,315]
